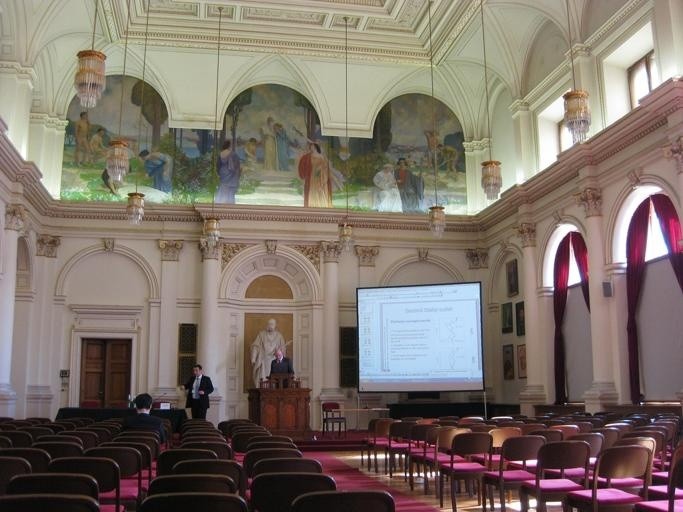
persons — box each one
[248,318,300,390]
[124,393,167,442]
[270,348,295,389]
[179,364,214,422]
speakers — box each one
[601,280,614,298]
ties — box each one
[278,360,280,364]
[195,378,199,396]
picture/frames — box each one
[501,259,527,381]
[178,323,197,389]
